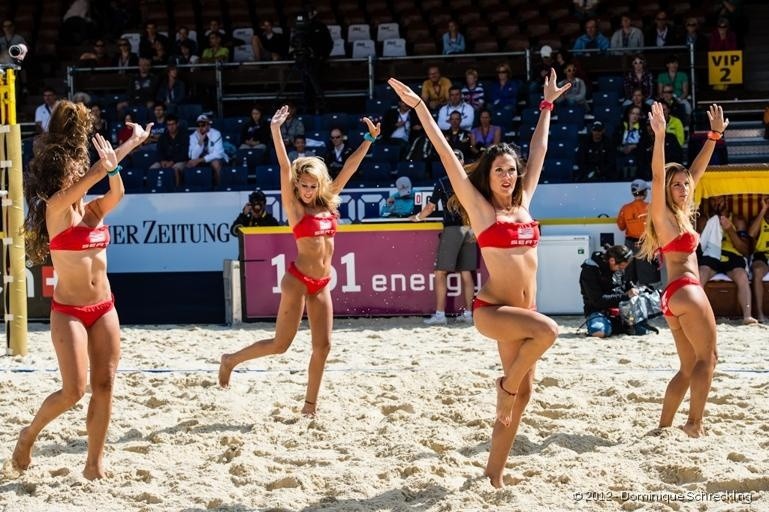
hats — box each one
[539,45,554,59]
[249,191,267,202]
[195,114,209,123]
[453,147,465,162]
[395,176,413,198]
[630,178,652,195]
[591,119,605,132]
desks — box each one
[235,222,489,322]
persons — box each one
[218,105,382,421]
[0,0,769,337]
[646,100,731,437]
[13,95,156,483]
[387,65,573,489]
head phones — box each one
[616,249,632,264]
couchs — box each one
[693,164,768,317]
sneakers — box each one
[455,310,474,325]
[424,312,448,326]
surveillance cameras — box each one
[8,43,27,63]
